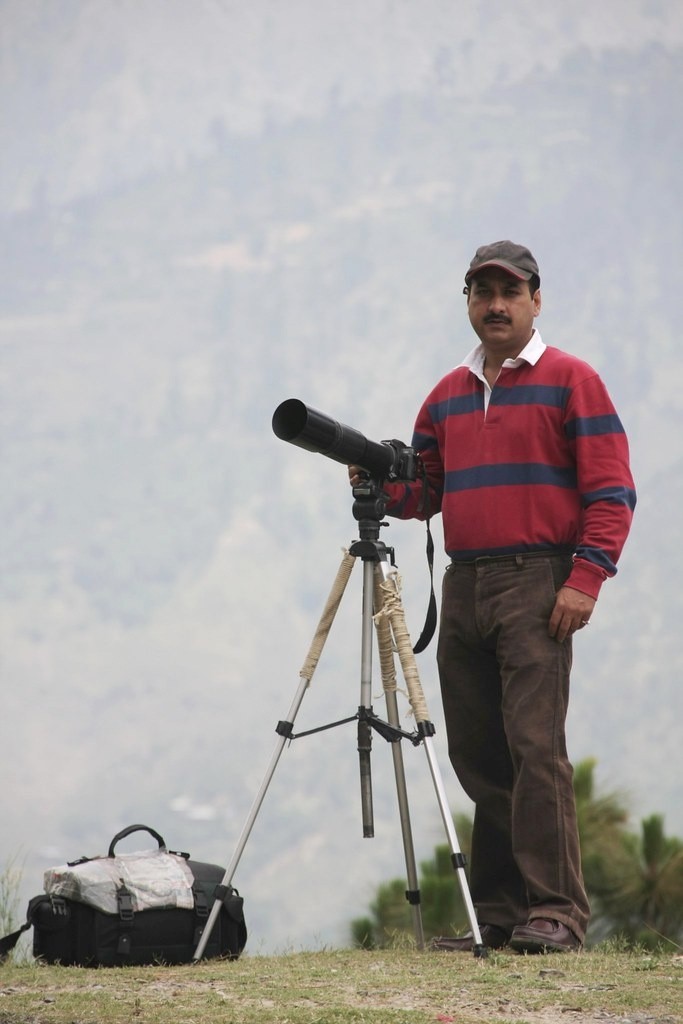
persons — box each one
[347,241,637,954]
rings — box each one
[581,620,590,625]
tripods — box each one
[190,479,489,965]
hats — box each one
[465,240,540,289]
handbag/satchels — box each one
[0,824,248,970]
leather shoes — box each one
[509,916,581,952]
[429,924,511,952]
